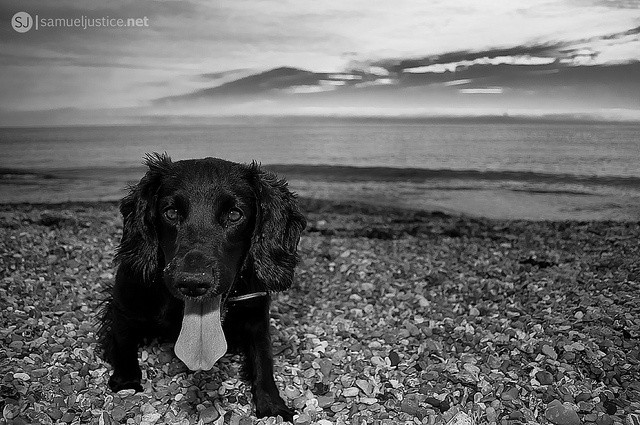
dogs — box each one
[92,151,307,424]
